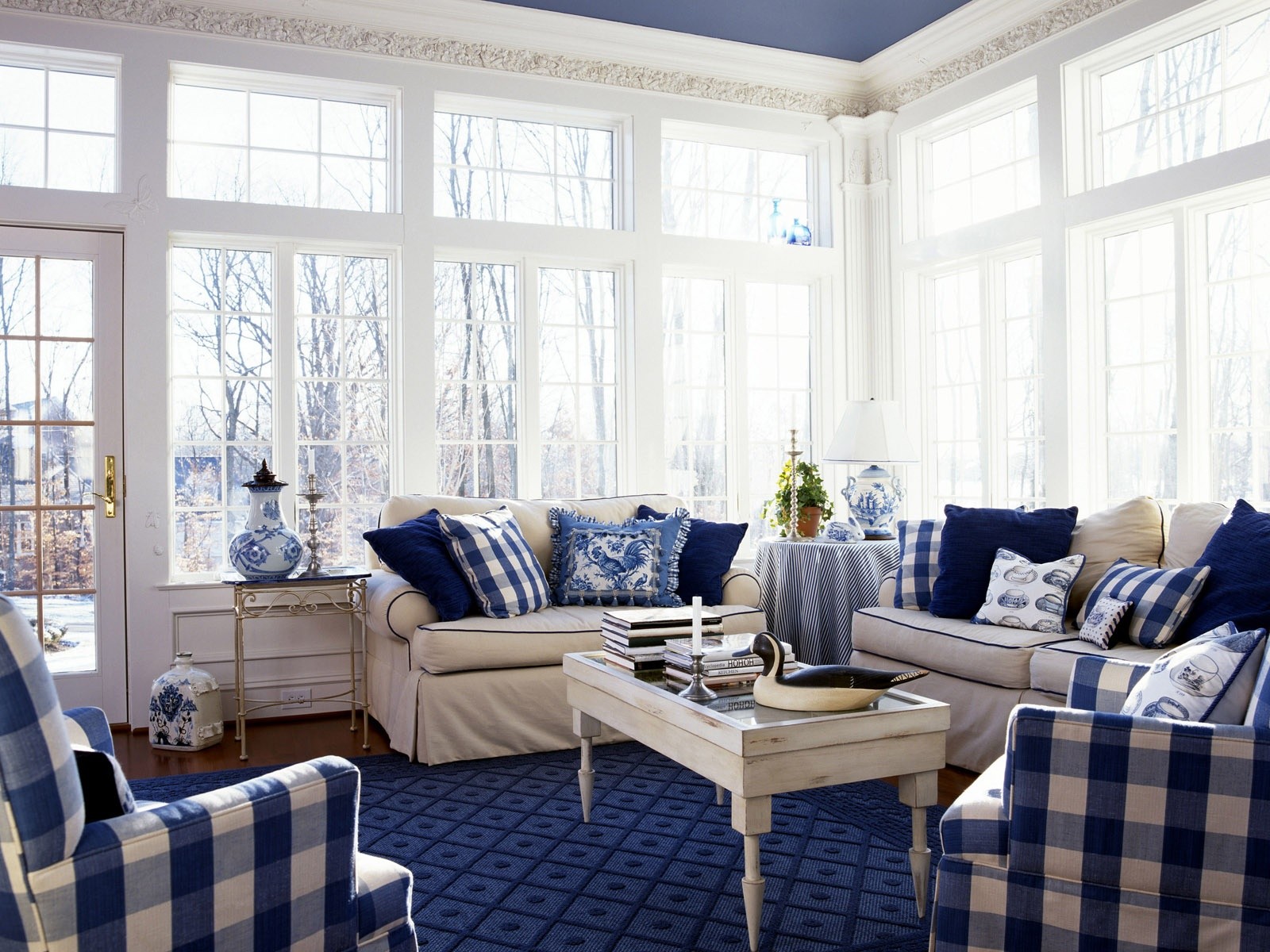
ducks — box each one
[732,632,930,711]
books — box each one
[600,602,799,691]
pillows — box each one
[638,505,748,606]
[360,507,476,621]
[897,493,1270,725]
[545,506,691,608]
[64,715,136,821]
[435,504,551,615]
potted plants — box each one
[762,459,834,539]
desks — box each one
[222,568,371,760]
[758,539,899,666]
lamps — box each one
[826,397,920,460]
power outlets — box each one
[282,688,312,709]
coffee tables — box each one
[561,649,951,952]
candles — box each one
[307,448,315,474]
[789,392,797,430]
[692,595,702,655]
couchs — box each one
[931,645,1269,952]
[850,562,1270,774]
[349,493,767,767]
[0,596,420,951]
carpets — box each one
[124,752,953,951]
